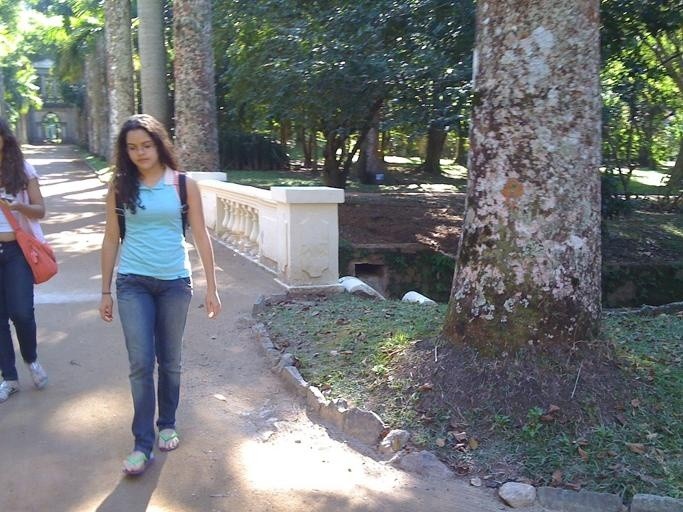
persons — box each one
[99,113,222,476]
[0,114,49,406]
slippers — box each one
[120,449,155,476]
[156,429,181,453]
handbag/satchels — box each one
[12,227,59,287]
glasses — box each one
[126,142,159,155]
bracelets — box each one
[101,292,111,295]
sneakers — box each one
[26,360,49,390]
[0,379,19,405]
